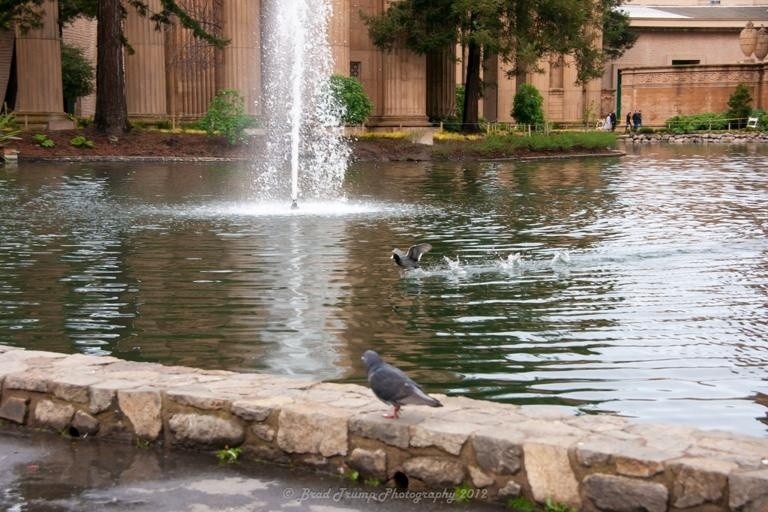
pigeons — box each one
[360,349,444,419]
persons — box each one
[625,109,645,133]
[596,110,616,132]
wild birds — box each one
[390,243,433,271]
